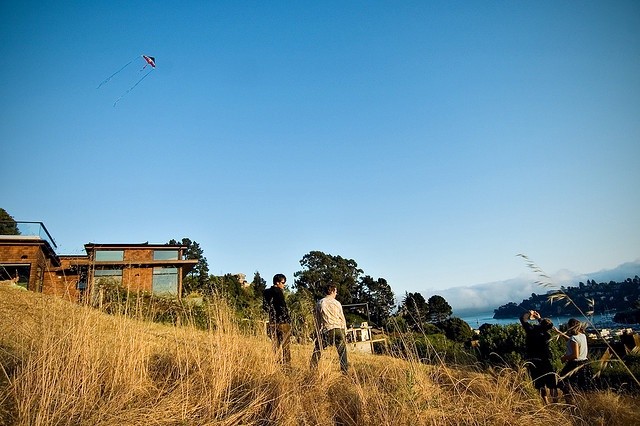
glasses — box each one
[280,281,285,284]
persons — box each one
[554,318,589,403]
[310,286,348,374]
[519,310,559,405]
[262,274,293,373]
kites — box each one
[96,54,156,107]
